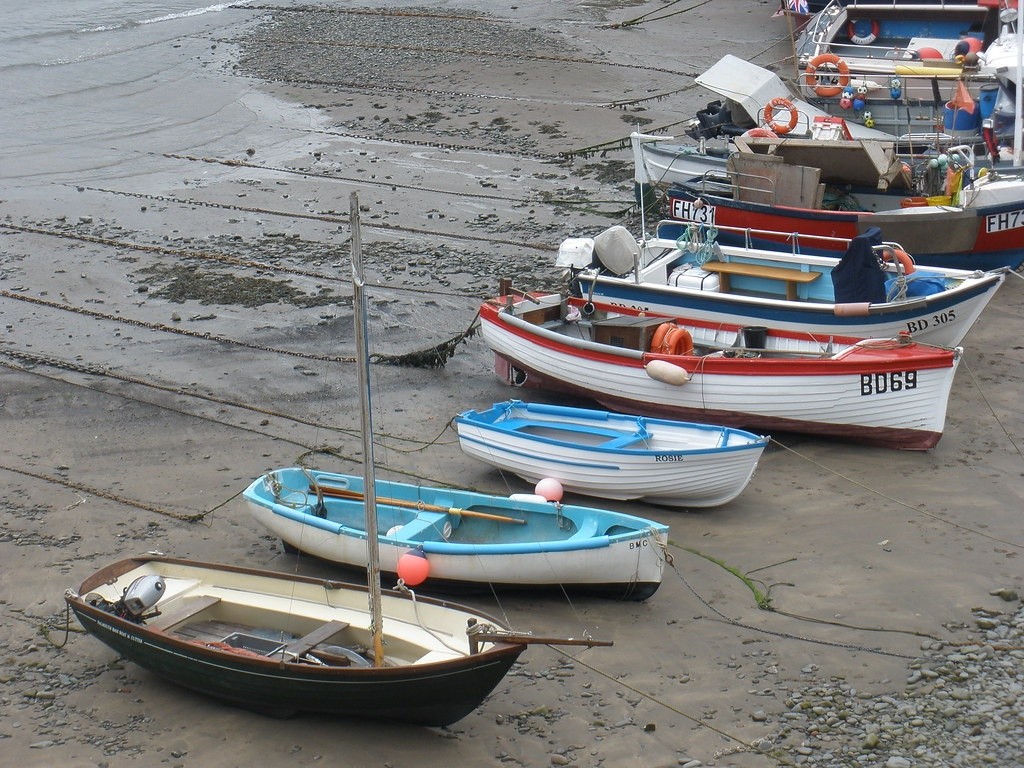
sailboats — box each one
[61,191,614,737]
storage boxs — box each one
[670,265,719,294]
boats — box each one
[556,124,1005,355]
[454,400,769,506]
[630,54,1024,273]
[244,467,668,603]
[481,291,964,450]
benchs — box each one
[538,317,822,361]
[269,619,350,662]
[701,260,823,300]
[386,512,448,541]
[148,594,222,631]
[597,432,651,450]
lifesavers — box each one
[738,96,799,139]
[805,53,851,97]
[649,320,694,356]
[846,19,879,46]
[883,247,914,276]
